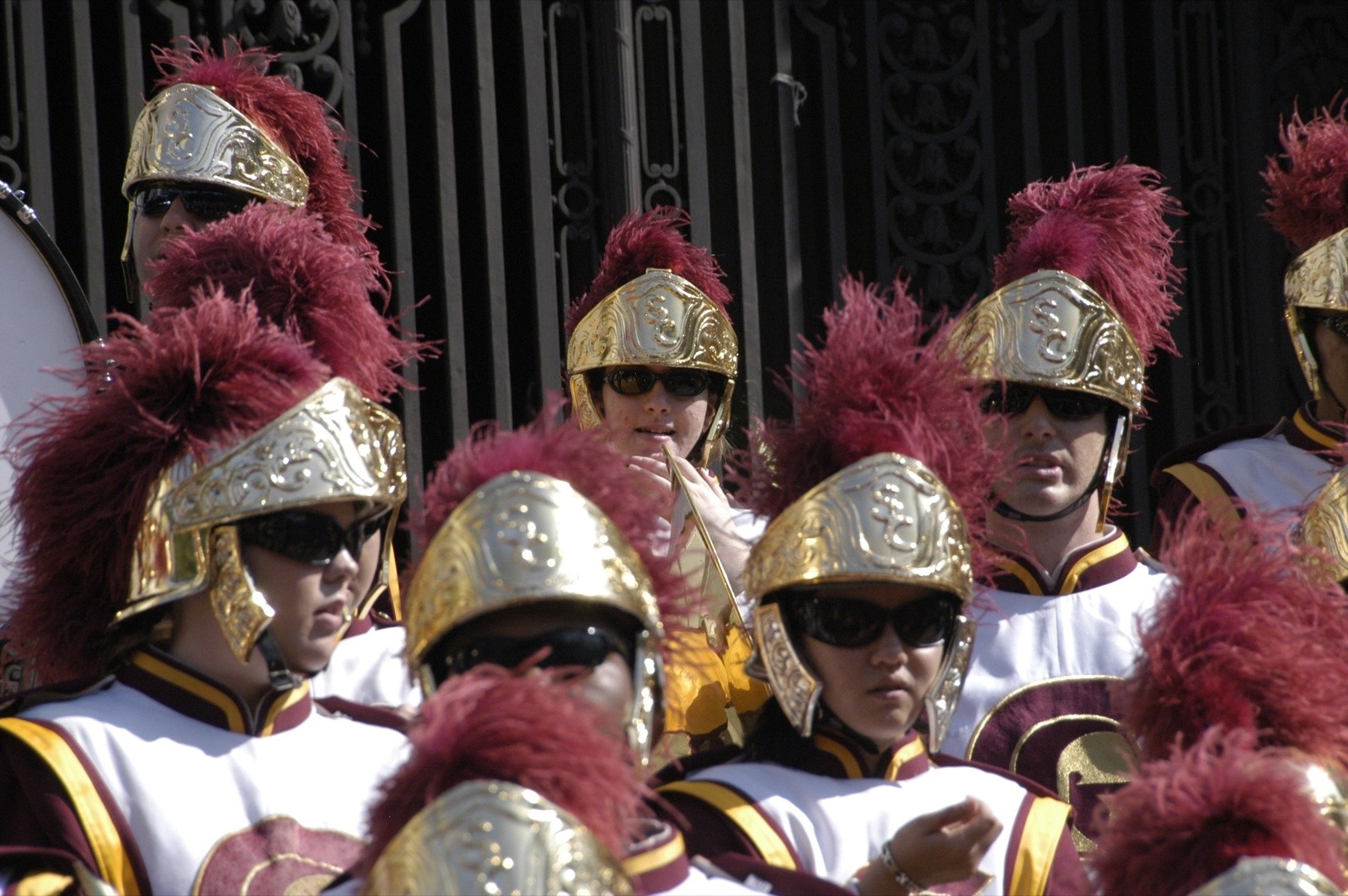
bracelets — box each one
[879,841,929,894]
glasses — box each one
[785,591,955,650]
[422,619,636,687]
[232,503,396,568]
[131,179,253,221]
[964,378,1116,422]
[1301,310,1347,343]
[601,363,725,401]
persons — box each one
[0,29,1348,896]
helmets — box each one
[9,286,409,689]
[1130,496,1348,832]
[936,154,1188,538]
[117,32,383,293]
[1290,458,1348,591]
[563,199,740,470]
[1254,85,1348,404]
[356,643,693,896]
[721,275,1037,756]
[1078,728,1348,895]
[396,394,706,773]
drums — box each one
[0,180,107,684]
